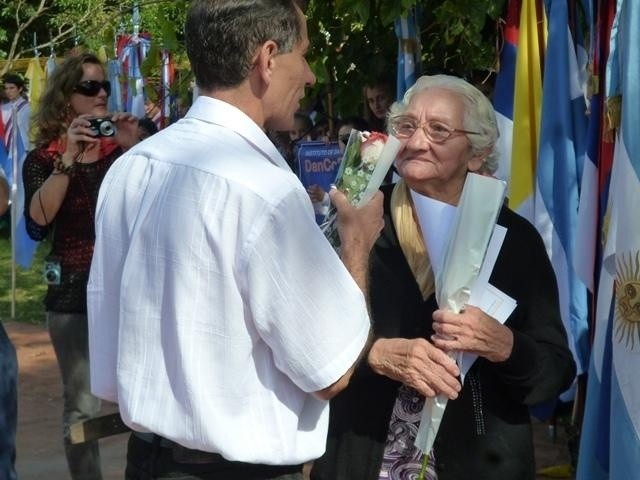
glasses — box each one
[69,78,113,99]
[388,114,487,145]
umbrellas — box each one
[391,2,420,101]
[19,18,198,138]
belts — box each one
[130,428,224,465]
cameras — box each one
[42,260,62,285]
[85,116,116,138]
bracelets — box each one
[51,159,77,180]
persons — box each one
[311,69,580,480]
[85,0,388,479]
[22,52,162,479]
[1,73,30,182]
